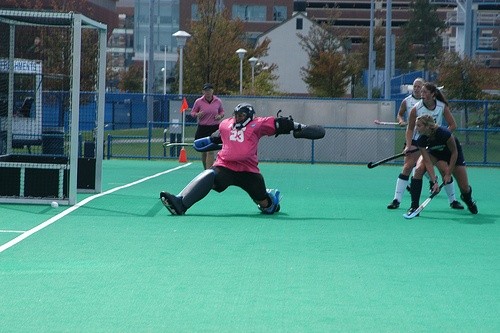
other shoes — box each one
[450,201,464,210]
[402,207,420,219]
[387,199,400,209]
[160,190,188,216]
[459,195,479,214]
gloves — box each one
[293,124,326,140]
[192,137,223,152]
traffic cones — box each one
[178,147,189,163]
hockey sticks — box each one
[163,141,193,148]
[402,180,446,219]
[368,149,420,169]
[375,119,404,125]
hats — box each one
[201,83,215,90]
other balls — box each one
[51,201,59,208]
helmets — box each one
[233,103,256,130]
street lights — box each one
[236,48,248,96]
[142,35,146,103]
[161,44,167,94]
[122,17,126,71]
[248,56,258,88]
[171,30,192,99]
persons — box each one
[160,103,325,215]
[387,77,478,217]
[190,83,225,169]
[0,95,34,118]
